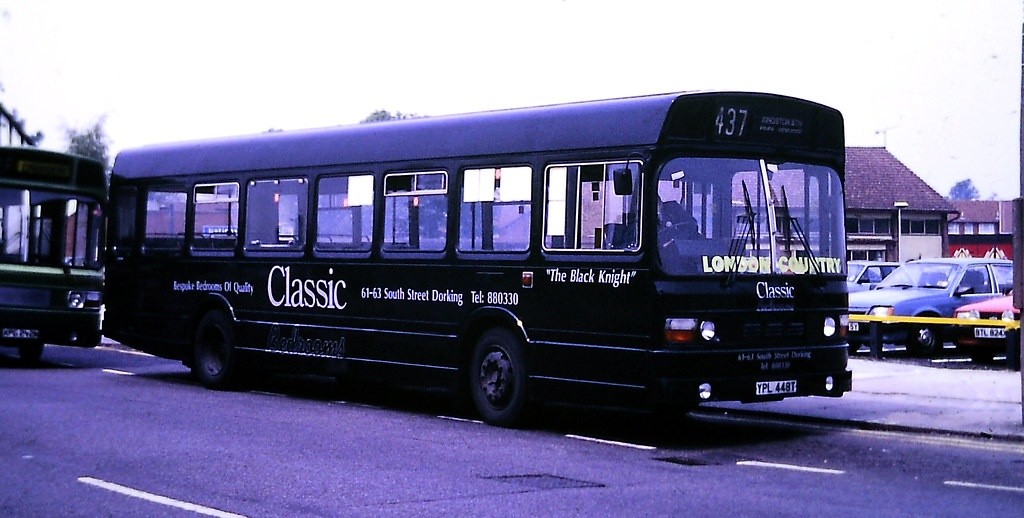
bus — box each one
[0,146,109,363]
[102,91,858,425]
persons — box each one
[656,194,699,235]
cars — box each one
[951,290,1019,365]
[845,257,1014,359]
[847,260,903,291]
